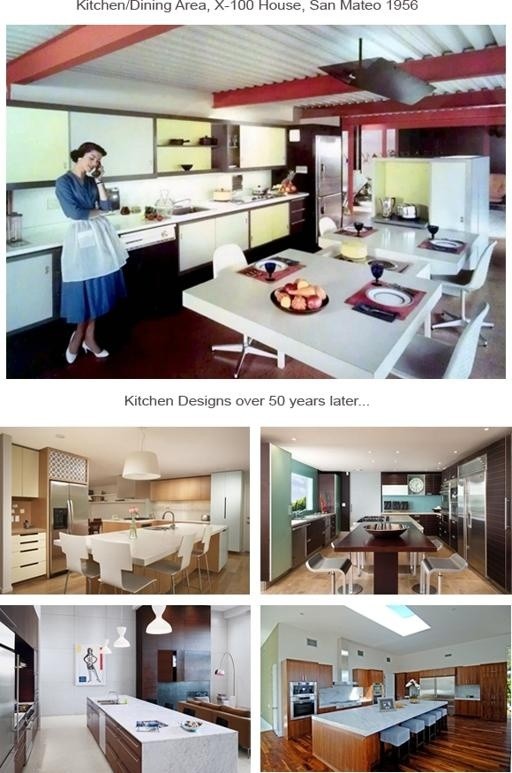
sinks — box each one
[173,206,209,216]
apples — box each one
[307,295,322,310]
[283,283,297,294]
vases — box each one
[129,521,138,539]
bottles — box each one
[170,139,190,145]
[7,212,23,243]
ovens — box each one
[290,681,318,720]
[26,709,33,764]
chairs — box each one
[306,522,468,593]
[58,526,215,594]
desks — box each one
[379,707,448,771]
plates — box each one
[365,287,414,307]
[254,259,288,272]
[181,720,203,732]
[430,239,463,249]
[269,289,330,314]
[344,227,367,233]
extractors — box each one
[332,638,359,686]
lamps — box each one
[123,428,160,481]
[318,38,436,107]
[99,604,174,654]
[215,652,235,695]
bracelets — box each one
[94,180,103,185]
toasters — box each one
[201,514,211,521]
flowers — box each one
[129,506,139,520]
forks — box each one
[361,305,393,316]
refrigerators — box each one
[0,621,19,772]
[287,135,344,243]
[49,481,88,576]
[457,454,487,577]
[420,676,455,716]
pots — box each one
[213,188,233,202]
[396,203,420,220]
[252,184,269,195]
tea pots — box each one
[379,197,396,217]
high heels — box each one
[82,341,109,358]
[65,331,78,364]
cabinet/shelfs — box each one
[280,659,383,741]
[86,695,239,773]
[9,442,50,585]
[89,469,244,554]
[262,442,340,590]
[380,435,512,594]
[0,606,39,772]
[395,662,507,723]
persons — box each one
[56,142,134,365]
[403,676,420,698]
[83,648,101,683]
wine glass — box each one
[371,259,385,286]
[354,221,364,237]
[427,223,439,239]
[265,262,275,281]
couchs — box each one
[178,697,251,759]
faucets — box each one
[173,198,192,204]
[162,510,175,530]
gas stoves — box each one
[15,703,33,713]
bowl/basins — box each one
[181,164,193,173]
[364,522,410,540]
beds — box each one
[181,224,483,379]
